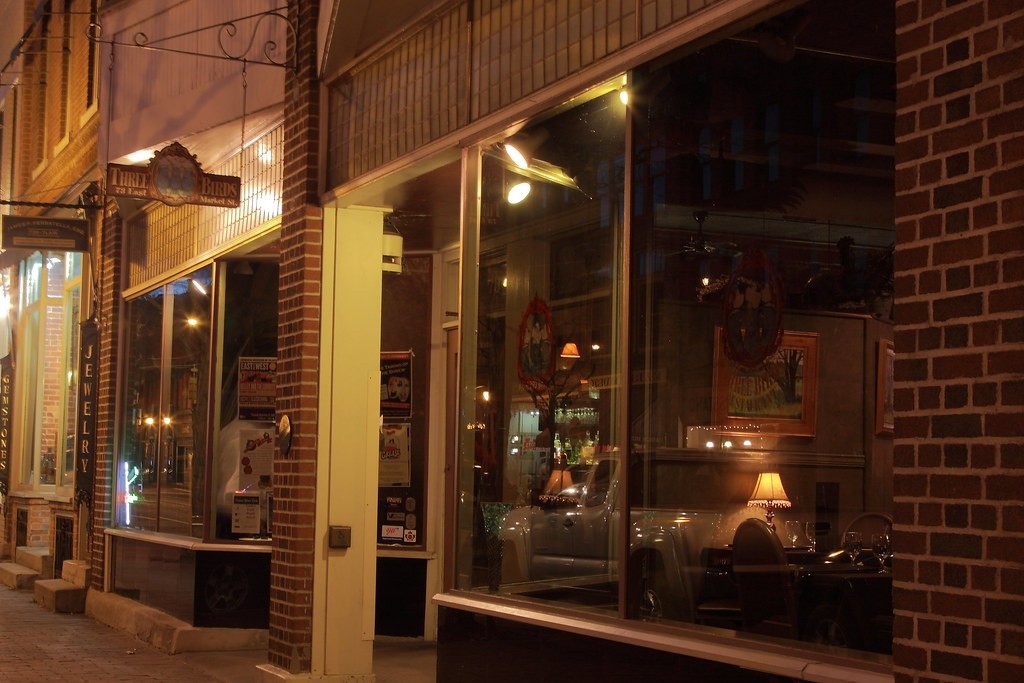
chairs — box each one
[841,512,894,551]
[732,519,800,642]
[677,523,741,631]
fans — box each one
[664,211,743,259]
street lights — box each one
[145,417,171,486]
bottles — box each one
[554,407,600,465]
[805,522,817,553]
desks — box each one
[789,563,892,645]
[699,544,819,604]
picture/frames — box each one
[875,337,894,436]
[711,326,820,438]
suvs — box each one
[490,442,866,617]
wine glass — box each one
[871,534,890,575]
[785,520,801,548]
[841,532,863,571]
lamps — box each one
[539,470,578,504]
[560,343,580,358]
[747,472,791,532]
[758,7,812,62]
[503,127,551,169]
[502,169,530,204]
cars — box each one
[126,462,154,484]
